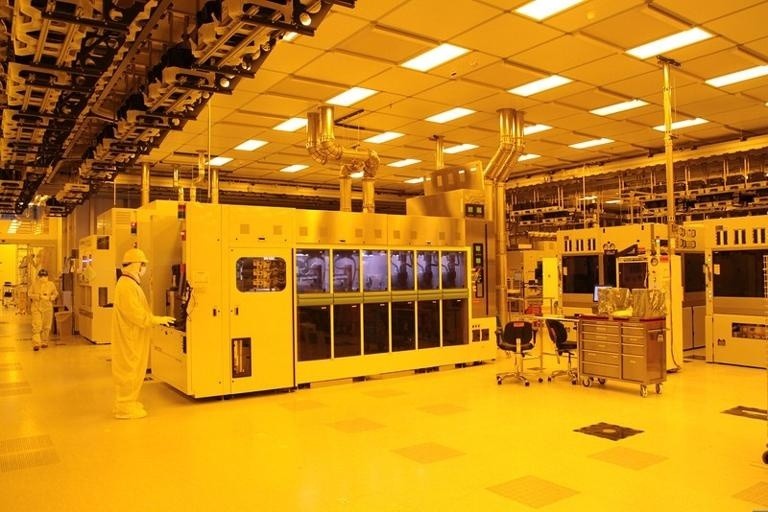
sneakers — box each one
[33,343,48,350]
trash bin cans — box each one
[55,311,73,345]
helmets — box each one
[122,248,150,264]
[38,269,48,276]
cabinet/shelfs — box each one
[574,312,667,398]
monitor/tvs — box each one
[592,285,614,302]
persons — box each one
[27,269,60,351]
[109,246,177,421]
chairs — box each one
[495,319,578,387]
